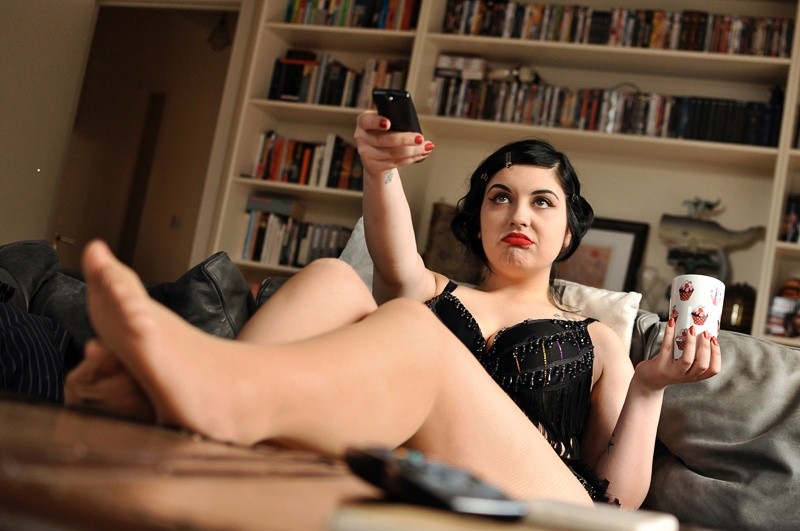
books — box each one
[230,194,353,267]
[431,77,771,147]
[428,0,796,58]
[284,0,421,30]
[250,125,365,190]
[267,49,408,109]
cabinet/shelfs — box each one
[187,0,800,350]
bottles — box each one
[720,191,800,340]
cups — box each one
[670,274,726,359]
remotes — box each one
[370,86,427,164]
[342,443,531,523]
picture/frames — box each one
[551,215,651,292]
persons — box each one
[64,89,726,510]
